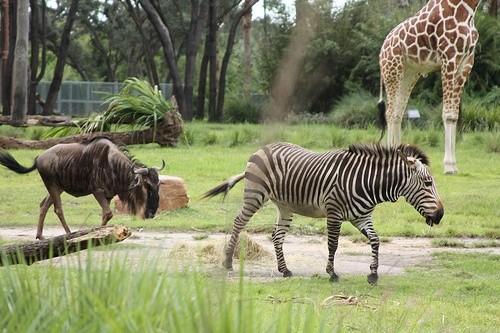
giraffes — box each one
[378,0,483,175]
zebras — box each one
[193,138,444,288]
